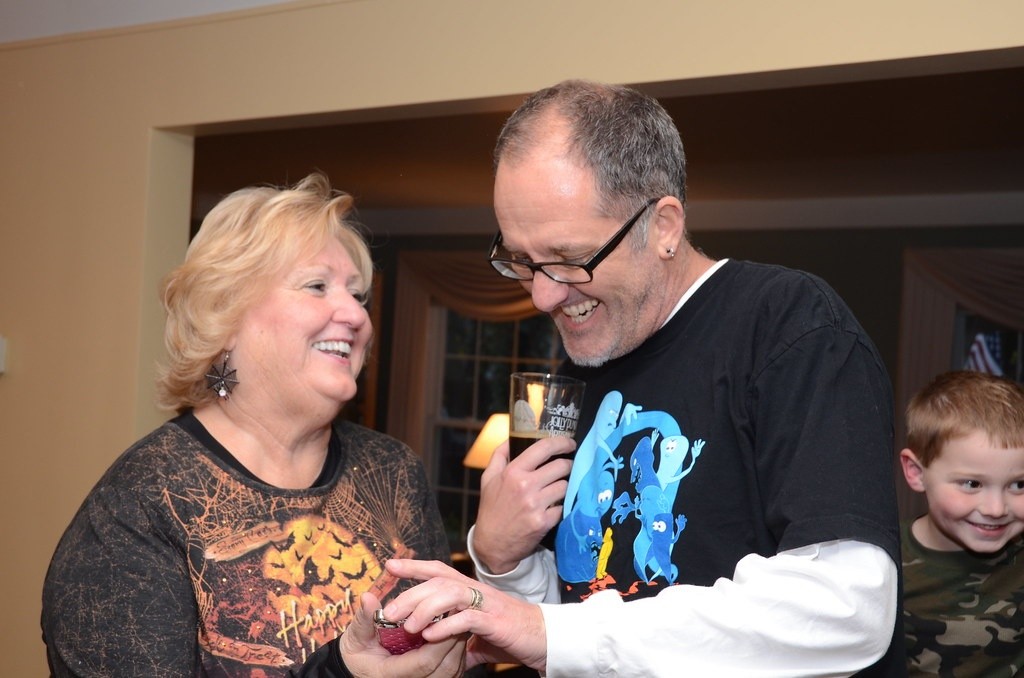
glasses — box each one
[487,197,657,285]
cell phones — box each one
[375,609,445,656]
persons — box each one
[40,174,528,678]
[896,372,1024,678]
[458,80,903,678]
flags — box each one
[958,331,1003,376]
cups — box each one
[509,371,586,509]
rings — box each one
[466,587,483,614]
